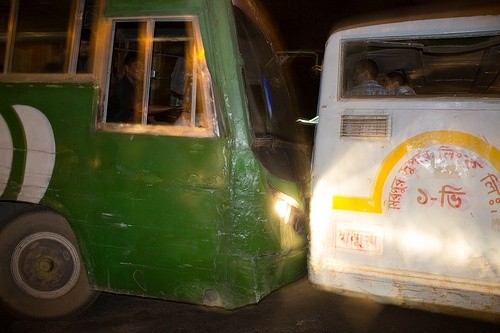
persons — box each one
[108,53,144,125]
[56,29,91,74]
[382,72,417,96]
[347,59,394,97]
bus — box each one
[1,1,310,321]
[307,11,499,323]
